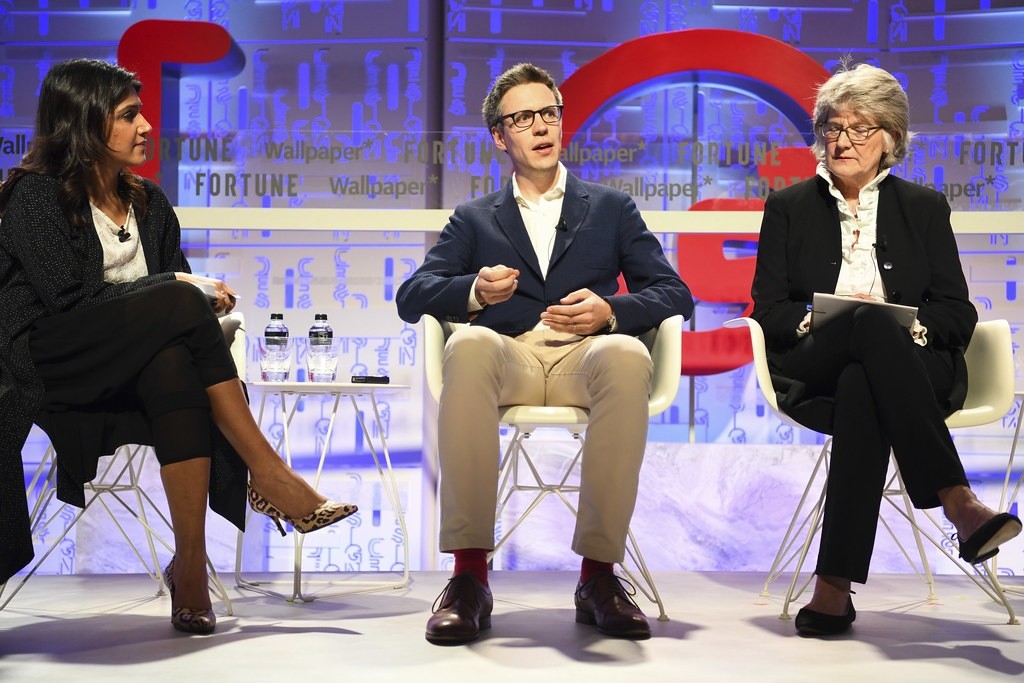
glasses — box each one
[819,122,883,142]
[491,104,564,128]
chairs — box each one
[0,311,247,617]
[419,313,687,620]
[723,317,1020,623]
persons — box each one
[396,62,694,646]
[749,62,1023,635]
[0,60,358,634]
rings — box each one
[220,291,226,294]
[572,324,576,331]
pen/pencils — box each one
[805,304,812,311]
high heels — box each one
[163,554,216,635]
[247,479,358,537]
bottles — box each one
[308,314,333,383]
[263,313,290,381]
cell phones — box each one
[352,375,389,384]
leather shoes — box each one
[574,571,651,637]
[425,573,493,642]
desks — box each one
[234,382,414,604]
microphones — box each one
[879,235,889,250]
[119,232,130,242]
[560,217,568,231]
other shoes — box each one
[795,594,856,635]
[951,512,1022,565]
